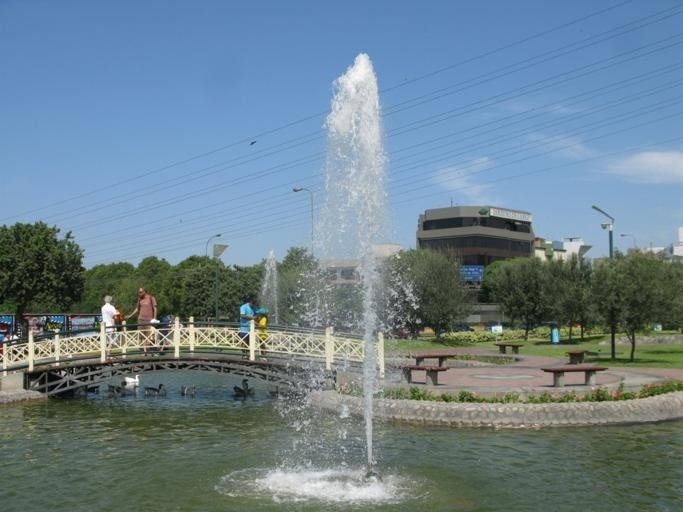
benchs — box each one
[386,349,456,385]
[539,365,609,388]
[495,342,523,354]
[565,349,588,363]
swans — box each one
[180,383,199,395]
[233,378,253,395]
[84,374,164,396]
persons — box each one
[92,286,175,360]
[1,318,56,361]
[238,297,270,362]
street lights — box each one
[591,205,615,358]
[204,234,229,327]
[292,187,314,255]
[621,233,637,248]
